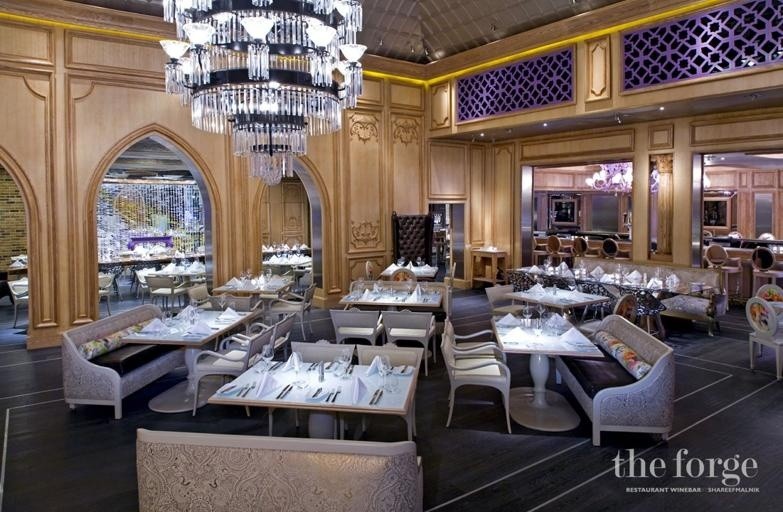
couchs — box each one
[61,302,188,419]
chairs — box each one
[7,279,30,329]
[0,270,15,306]
[98,230,783,512]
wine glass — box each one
[354,256,440,304]
[516,254,675,341]
[162,255,199,275]
[267,240,301,263]
[237,269,272,287]
[218,344,413,406]
[158,293,238,326]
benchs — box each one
[8,266,28,279]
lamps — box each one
[584,162,660,194]
[160,0,368,186]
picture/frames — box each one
[703,195,733,233]
[550,195,580,228]
